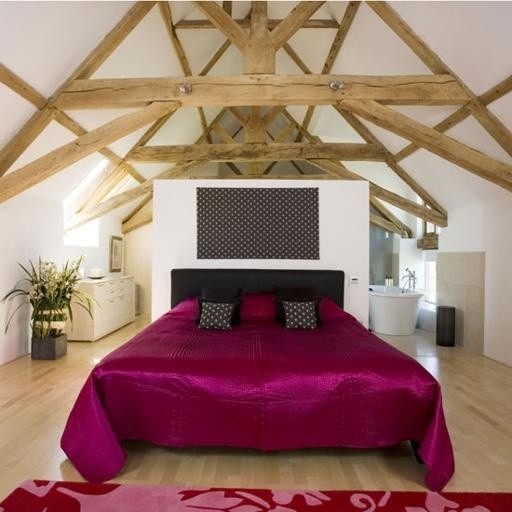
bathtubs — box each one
[371,283,426,336]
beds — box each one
[61,269,455,491]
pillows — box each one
[197,295,240,332]
[280,300,317,330]
[196,285,243,325]
[276,296,321,329]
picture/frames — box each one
[110,236,123,272]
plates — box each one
[87,275,105,279]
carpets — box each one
[2,479,511,511]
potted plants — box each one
[0,253,104,361]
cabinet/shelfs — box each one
[65,274,136,343]
